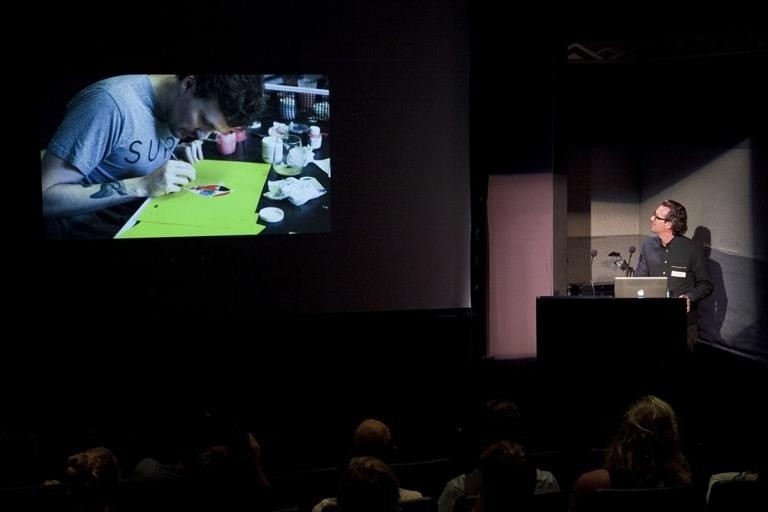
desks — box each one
[112,119,330,238]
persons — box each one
[313,418,424,512]
[41,428,271,507]
[438,398,558,512]
[573,397,693,510]
[40,71,264,223]
[633,198,716,338]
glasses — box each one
[654,211,667,220]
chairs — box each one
[24,454,767,512]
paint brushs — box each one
[159,137,191,182]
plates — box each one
[268,127,284,139]
[290,124,309,134]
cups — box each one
[261,137,284,164]
[220,132,236,154]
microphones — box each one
[589,248,597,296]
[625,246,635,277]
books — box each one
[112,157,273,242]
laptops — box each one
[614,277,668,298]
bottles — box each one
[306,125,323,149]
[272,135,304,176]
